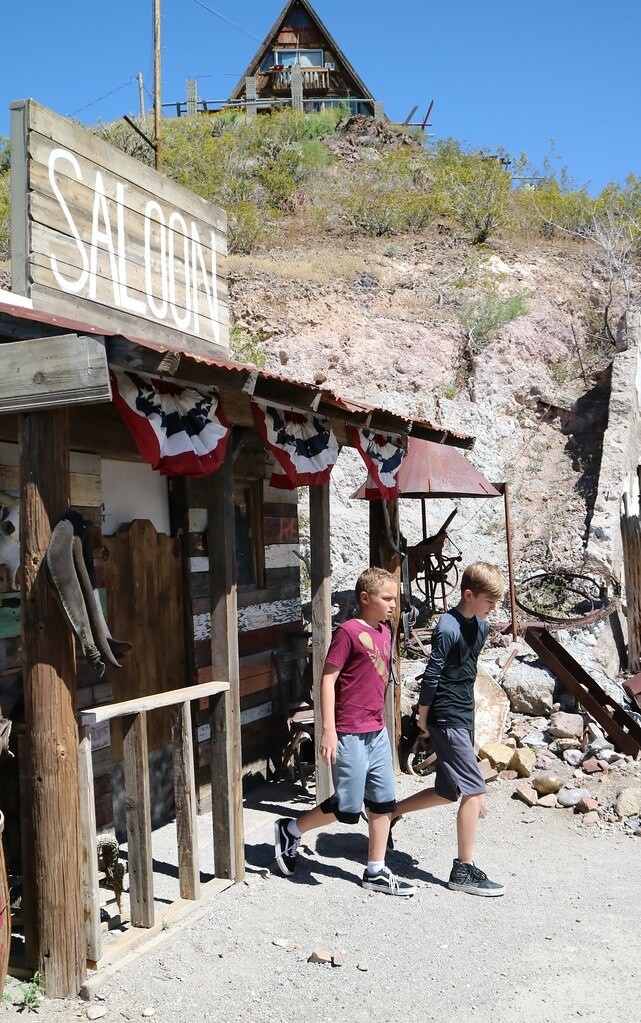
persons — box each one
[385,562,504,896]
[275,567,415,895]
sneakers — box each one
[448,859,506,897]
[362,866,414,895]
[362,805,399,853]
[274,818,301,876]
